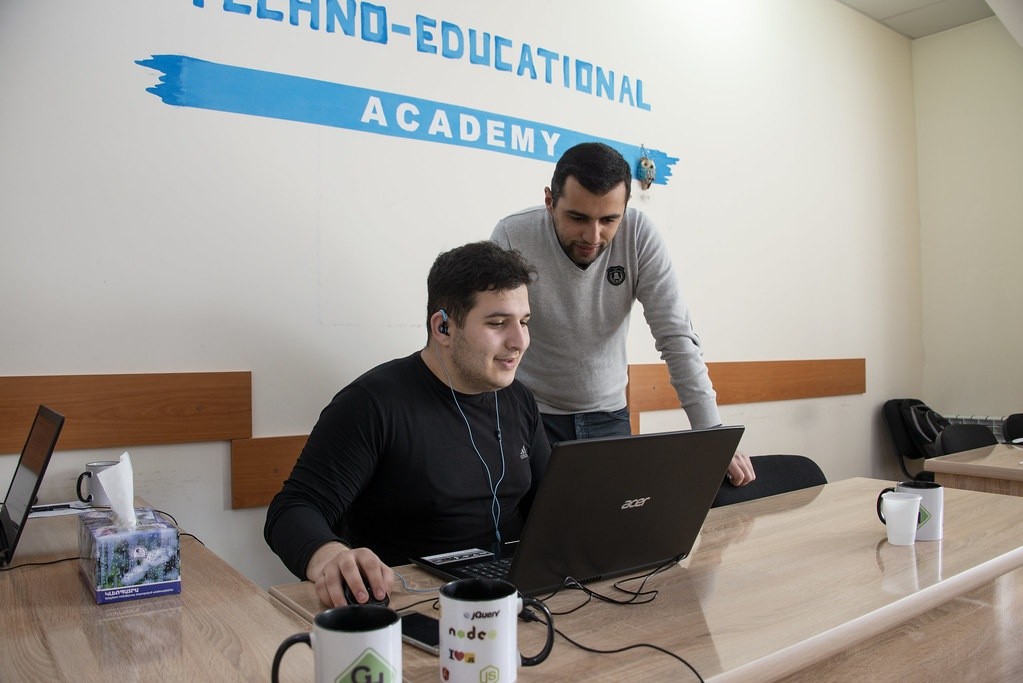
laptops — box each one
[0,403,65,566]
[407,425,745,600]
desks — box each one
[0,443,1023,683]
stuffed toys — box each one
[637,158,656,190]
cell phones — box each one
[397,610,440,656]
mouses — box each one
[342,574,389,608]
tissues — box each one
[78,451,182,607]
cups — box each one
[876,480,945,541]
[881,492,923,547]
[76,461,120,507]
[271,604,403,683]
[437,578,555,683]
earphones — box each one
[438,320,448,335]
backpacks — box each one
[898,399,952,459]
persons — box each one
[489,142,758,488]
[262,241,552,611]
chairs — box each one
[1002,412,1023,445]
[941,425,1000,456]
[709,456,829,505]
[882,398,951,482]
